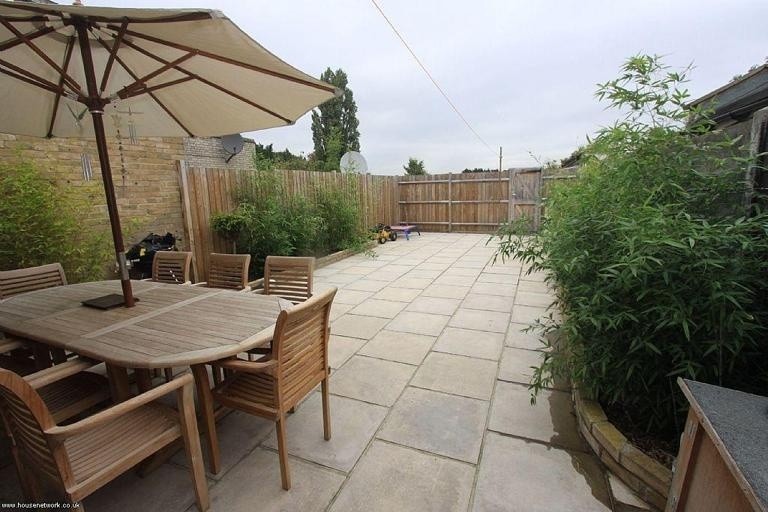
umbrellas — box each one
[0,0,344,396]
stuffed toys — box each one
[371,222,396,245]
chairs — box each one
[0,249,338,511]
